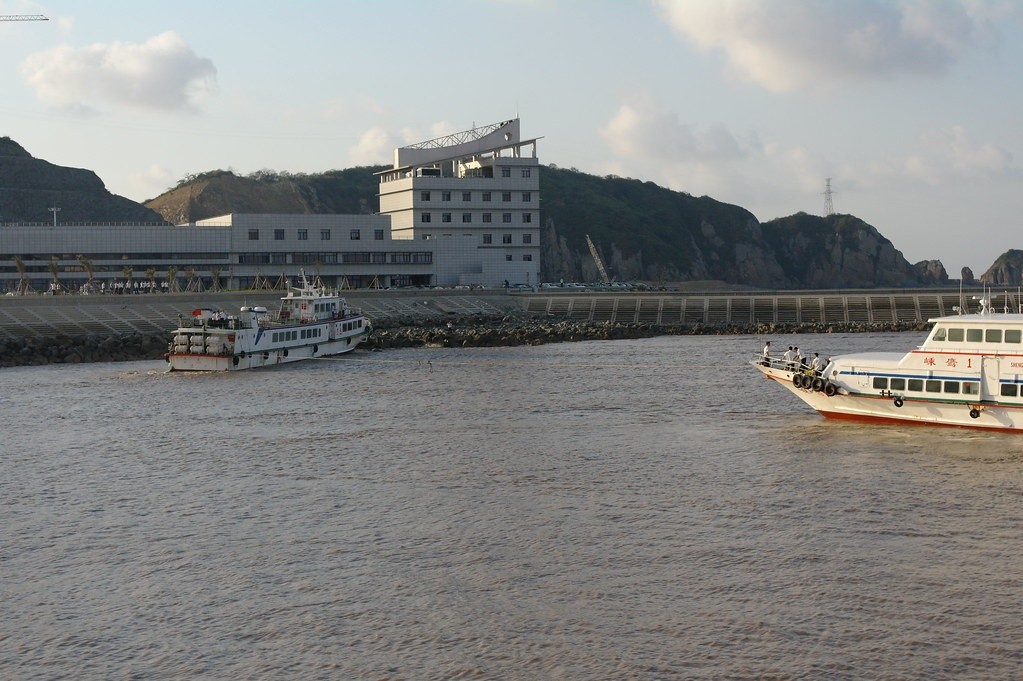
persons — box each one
[763,341,771,367]
[781,347,797,371]
[341,303,346,318]
[504,280,509,288]
[792,345,806,374]
[49,279,170,295]
[209,309,228,329]
[808,352,822,375]
[331,304,334,319]
[560,277,563,287]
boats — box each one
[163,267,374,372]
[748,276,1023,434]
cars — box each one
[372,281,674,294]
